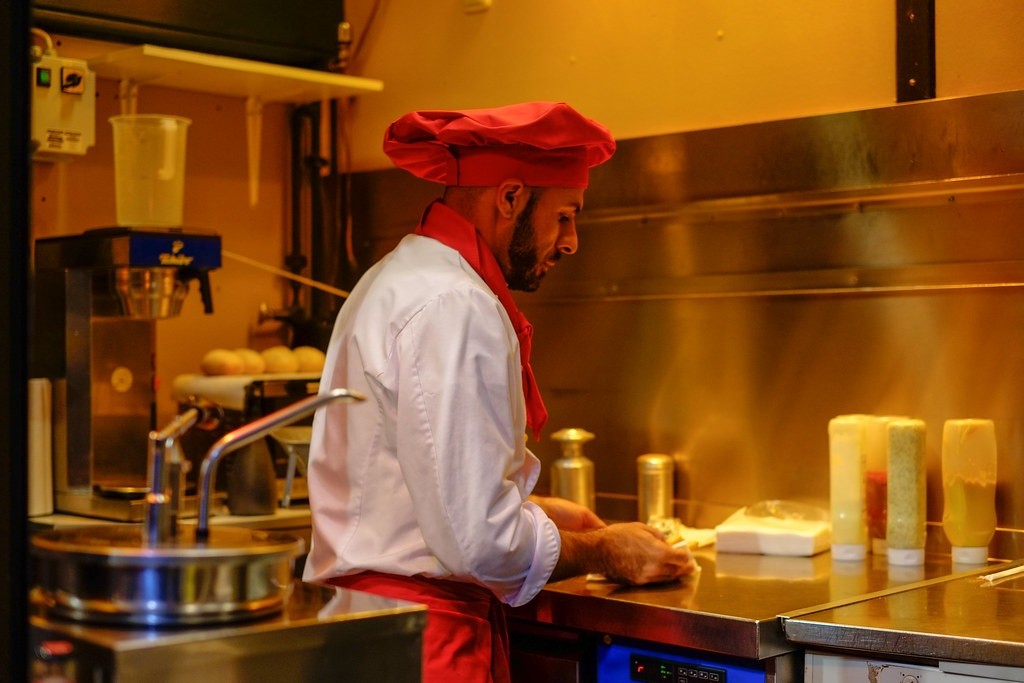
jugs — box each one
[109,115,190,226]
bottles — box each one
[550,428,596,512]
[941,418,998,565]
[637,453,673,523]
[827,414,928,565]
[228,435,277,517]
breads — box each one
[202,346,328,374]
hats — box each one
[383,98,617,188]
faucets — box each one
[142,397,225,539]
[196,388,370,544]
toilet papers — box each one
[26,377,54,518]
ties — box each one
[411,199,548,444]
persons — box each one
[301,102,692,683]
